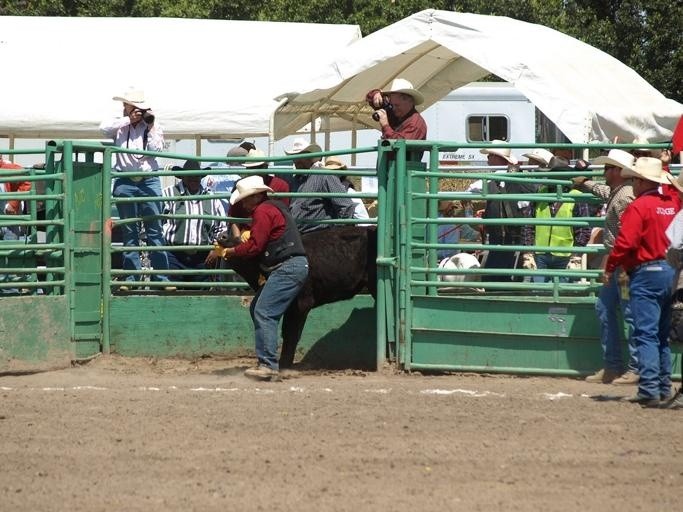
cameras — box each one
[373,101,393,121]
[133,108,154,124]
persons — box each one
[0,158,47,293]
[366,77,429,166]
[95,89,180,292]
[117,138,369,290]
[599,154,683,409]
[203,172,309,381]
[665,203,683,278]
[569,149,638,385]
[438,141,683,293]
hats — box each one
[627,137,654,151]
[438,200,455,211]
[479,141,592,178]
[112,86,152,109]
[593,148,635,171]
[382,79,425,105]
[172,142,347,205]
[620,157,675,185]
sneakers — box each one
[244,365,280,382]
[162,286,176,291]
[623,388,683,409]
[120,283,133,291]
[585,367,623,383]
[611,371,641,385]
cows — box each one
[215,223,378,379]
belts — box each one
[177,253,209,264]
[632,259,667,270]
[120,174,157,181]
[273,252,306,265]
